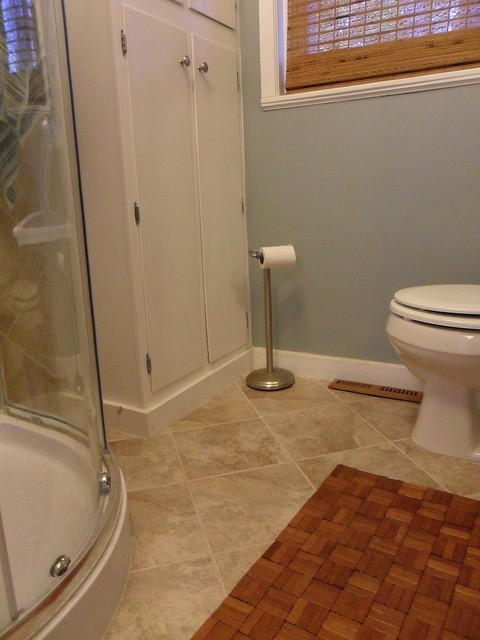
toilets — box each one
[385,284,480,463]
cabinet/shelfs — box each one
[37,0,254,435]
